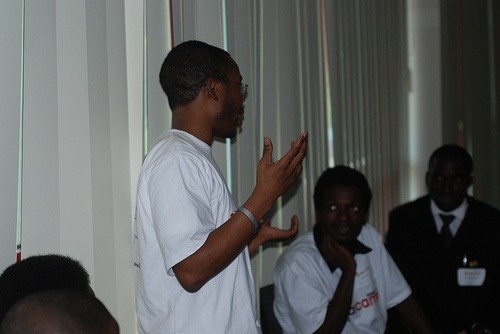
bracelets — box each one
[237,206,259,234]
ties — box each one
[440,214,455,239]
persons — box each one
[385,144,500,334]
[273,165,435,334]
[0,254,119,334]
[132,40,308,334]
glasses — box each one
[218,78,249,103]
